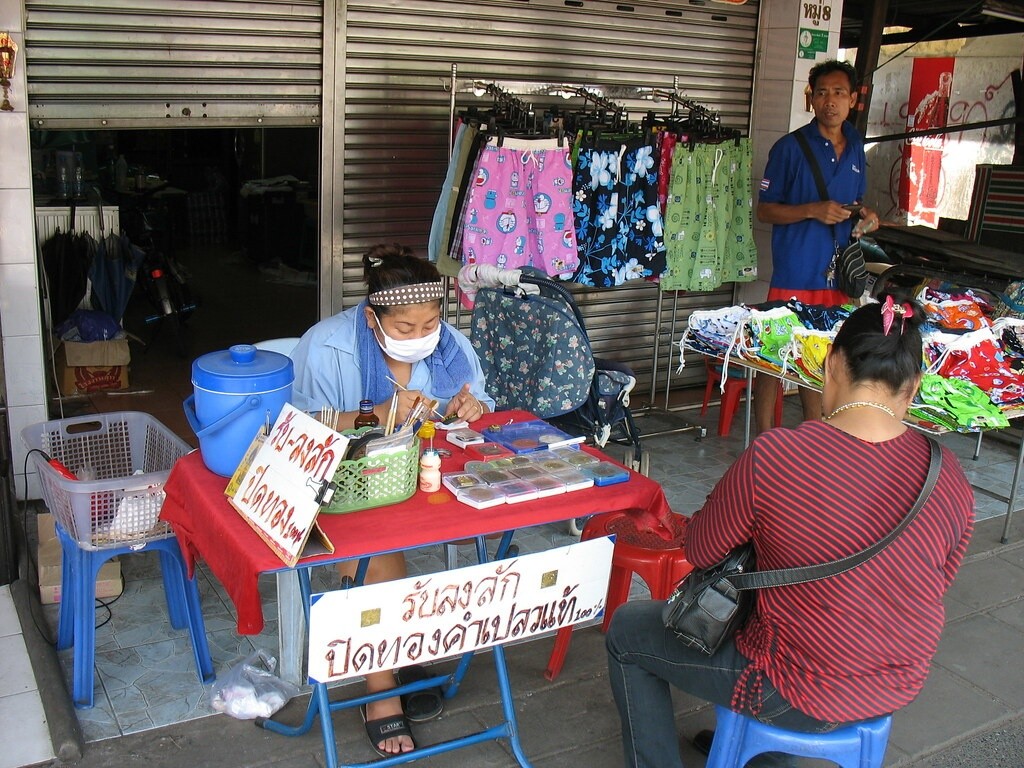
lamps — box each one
[981,4,1024,23]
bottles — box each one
[116,154,127,178]
[136,164,145,193]
[417,420,436,439]
[419,447,442,493]
[104,145,116,189]
[354,400,380,430]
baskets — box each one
[21,412,193,551]
[321,426,420,513]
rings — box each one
[873,220,875,224]
[473,405,478,411]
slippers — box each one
[398,665,444,721]
[359,704,418,763]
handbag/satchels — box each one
[661,539,757,657]
[838,237,869,299]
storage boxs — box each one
[442,419,630,510]
[47,331,147,396]
[37,512,123,605]
[20,410,194,552]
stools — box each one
[704,705,892,768]
[56,521,216,710]
[544,510,694,681]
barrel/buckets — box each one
[182,344,295,479]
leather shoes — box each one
[694,730,797,768]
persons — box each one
[286,243,496,765]
[755,59,881,433]
[605,290,977,768]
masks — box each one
[373,311,440,363]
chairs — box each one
[700,355,783,438]
[252,337,310,687]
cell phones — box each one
[841,205,864,217]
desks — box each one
[158,410,679,768]
[245,183,318,268]
[672,341,1024,545]
[105,177,195,251]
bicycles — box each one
[121,179,199,363]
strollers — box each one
[456,263,650,481]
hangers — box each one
[458,84,741,152]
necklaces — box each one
[824,401,897,422]
